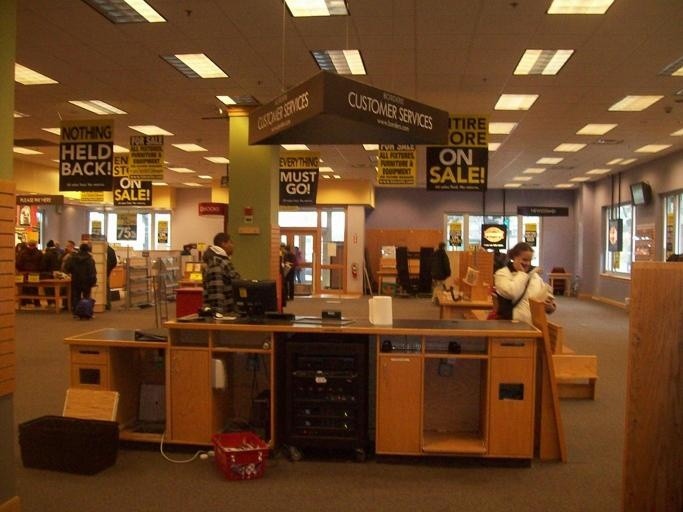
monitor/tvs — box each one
[630,182,650,206]
[231,279,278,323]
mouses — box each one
[215,312,224,318]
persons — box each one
[494,243,556,324]
[280,242,301,307]
[15,240,116,318]
[431,243,450,303]
[202,233,237,312]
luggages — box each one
[77,285,98,319]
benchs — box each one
[546,317,598,401]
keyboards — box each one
[239,312,295,320]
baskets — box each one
[212,432,270,480]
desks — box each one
[64,312,544,467]
[436,288,492,321]
[548,272,571,296]
[16,279,73,317]
